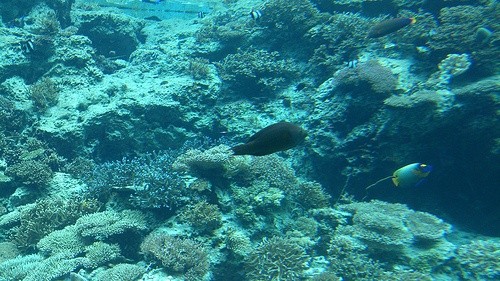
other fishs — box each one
[392,163,435,190]
[2,15,38,55]
[195,11,207,18]
[250,8,265,20]
[224,122,309,156]
[470,27,492,47]
[362,17,418,39]
[140,0,165,5]
